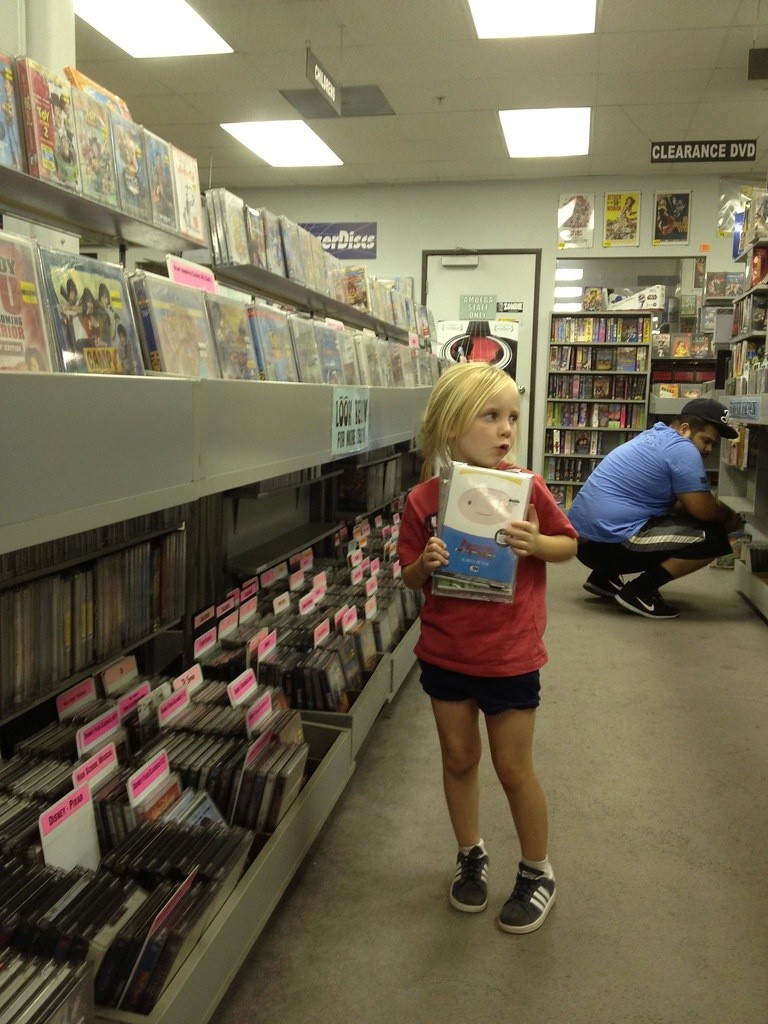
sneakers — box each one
[498,861,556,934]
[583,570,624,601]
[614,581,680,619]
[449,846,489,912]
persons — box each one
[110,320,133,372]
[62,280,78,316]
[98,284,117,340]
[397,361,578,934]
[568,399,747,619]
[80,288,103,338]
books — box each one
[0,54,440,388]
[724,295,768,394]
[433,463,532,601]
[653,333,716,399]
[0,496,423,1024]
[546,316,650,508]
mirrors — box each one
[552,255,706,334]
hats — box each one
[681,398,738,439]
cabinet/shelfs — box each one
[0,165,435,1023]
[543,310,654,520]
[648,237,767,626]
